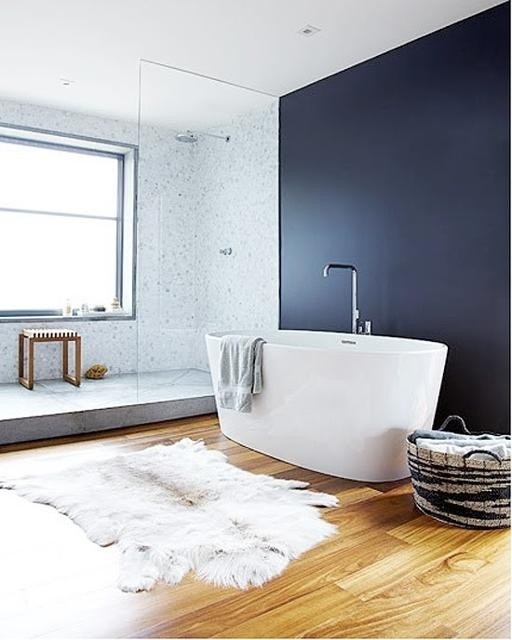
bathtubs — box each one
[205,330,448,483]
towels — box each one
[214,333,267,414]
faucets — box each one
[323,264,357,332]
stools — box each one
[18,328,82,391]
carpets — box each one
[0,435,343,596]
